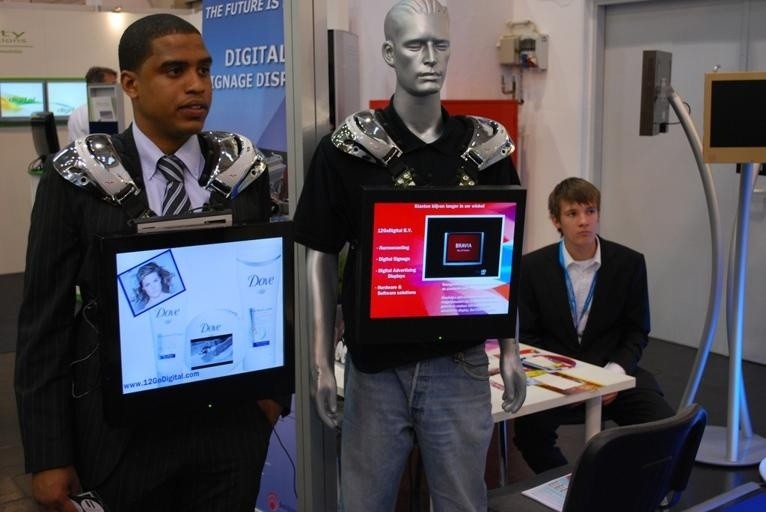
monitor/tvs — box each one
[31,112,60,162]
[46,77,87,120]
[356,185,528,344]
[100,220,296,435]
[639,49,673,135]
[703,71,765,165]
[0,78,48,125]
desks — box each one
[326,337,636,512]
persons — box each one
[513,177,677,475]
[292,0,527,512]
[68,67,117,144]
[14,13,292,512]
[133,262,173,309]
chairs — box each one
[489,406,708,512]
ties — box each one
[156,156,193,216]
